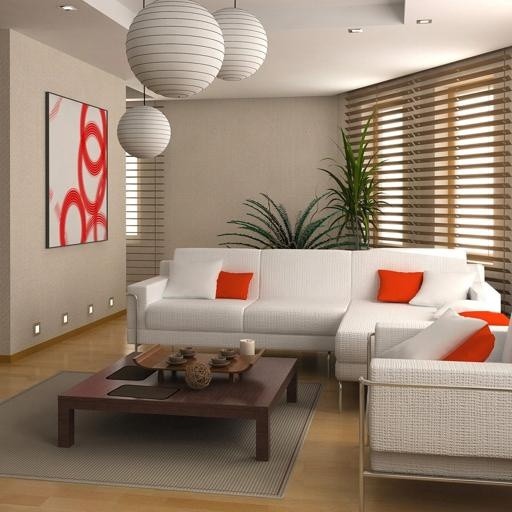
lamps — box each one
[211,0,268,81]
[117,0,171,159]
[126,0,225,97]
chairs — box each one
[358,321,512,512]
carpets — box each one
[0,371,322,499]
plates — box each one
[218,353,238,360]
[208,361,231,368]
[184,351,198,358]
[167,359,187,365]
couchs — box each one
[127,248,502,413]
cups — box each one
[168,354,184,362]
[212,357,226,363]
[220,349,235,355]
[179,348,194,354]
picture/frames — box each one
[45,92,108,248]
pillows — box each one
[377,270,510,362]
[216,271,253,300]
[162,260,222,300]
